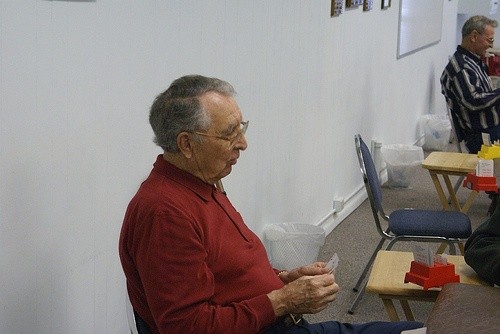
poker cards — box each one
[325,252,340,273]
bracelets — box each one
[277,271,288,277]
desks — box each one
[424,281,500,334]
[422,151,478,256]
[365,250,491,322]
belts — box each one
[283,313,303,327]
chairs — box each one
[348,135,473,315]
[126,292,152,334]
[445,108,465,206]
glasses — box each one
[187,121,249,150]
[478,32,496,43]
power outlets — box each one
[333,198,345,212]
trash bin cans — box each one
[382,143,424,190]
[263,221,326,272]
[420,115,452,152]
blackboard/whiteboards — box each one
[396,0,444,60]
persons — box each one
[118,74,426,334]
[440,15,500,200]
[464,204,500,288]
